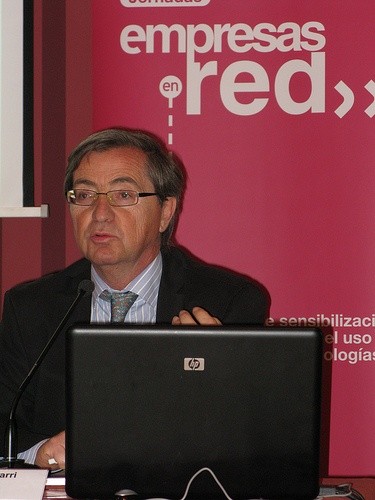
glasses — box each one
[66,189,169,207]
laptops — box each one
[65,323,323,500]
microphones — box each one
[0,280,95,469]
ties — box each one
[98,290,139,323]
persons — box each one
[0,126,270,471]
